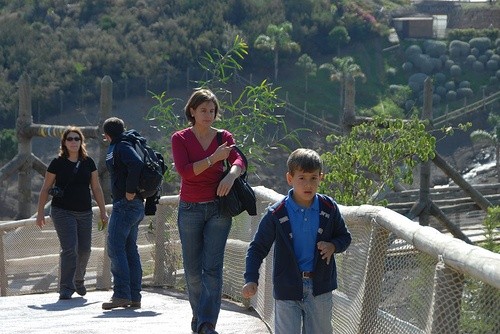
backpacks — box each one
[128,129,166,198]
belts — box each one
[301,272,312,278]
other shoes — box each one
[130,300,140,308]
[198,323,218,334]
[191,317,197,332]
[77,285,86,295]
[60,287,71,299]
[102,295,131,308]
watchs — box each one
[206,157,214,168]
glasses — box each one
[66,136,81,142]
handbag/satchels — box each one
[216,129,258,217]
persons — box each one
[171,89,245,333]
[37,127,109,301]
[242,147,351,334]
[102,117,144,310]
